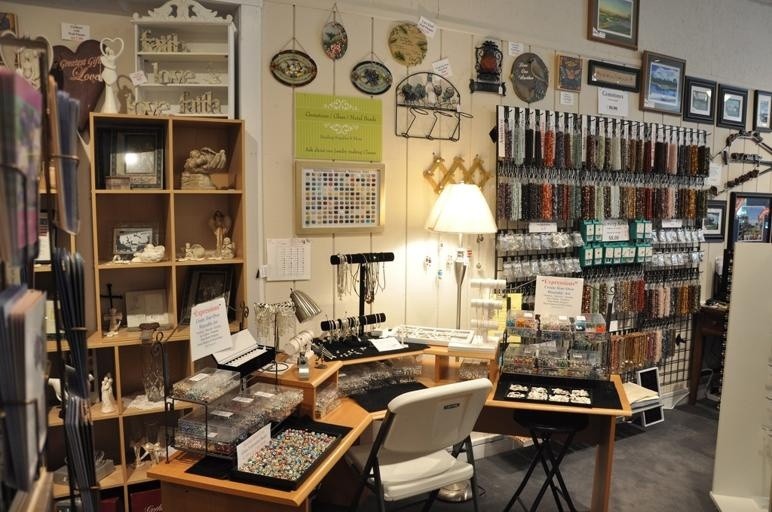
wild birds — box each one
[527,58,548,87]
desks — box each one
[148,350,632,510]
[687,305,728,406]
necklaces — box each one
[326,312,384,346]
[336,253,390,306]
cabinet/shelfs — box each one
[83,113,252,339]
[43,331,218,510]
[129,1,238,119]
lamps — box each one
[424,182,499,504]
[289,287,323,324]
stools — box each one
[508,407,587,510]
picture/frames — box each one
[701,200,726,241]
[682,75,715,124]
[585,0,641,53]
[179,266,233,327]
[728,192,772,248]
[100,219,164,265]
[752,88,772,132]
[637,48,686,116]
[587,60,641,93]
[108,124,166,188]
[717,83,747,130]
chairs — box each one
[348,375,492,512]
